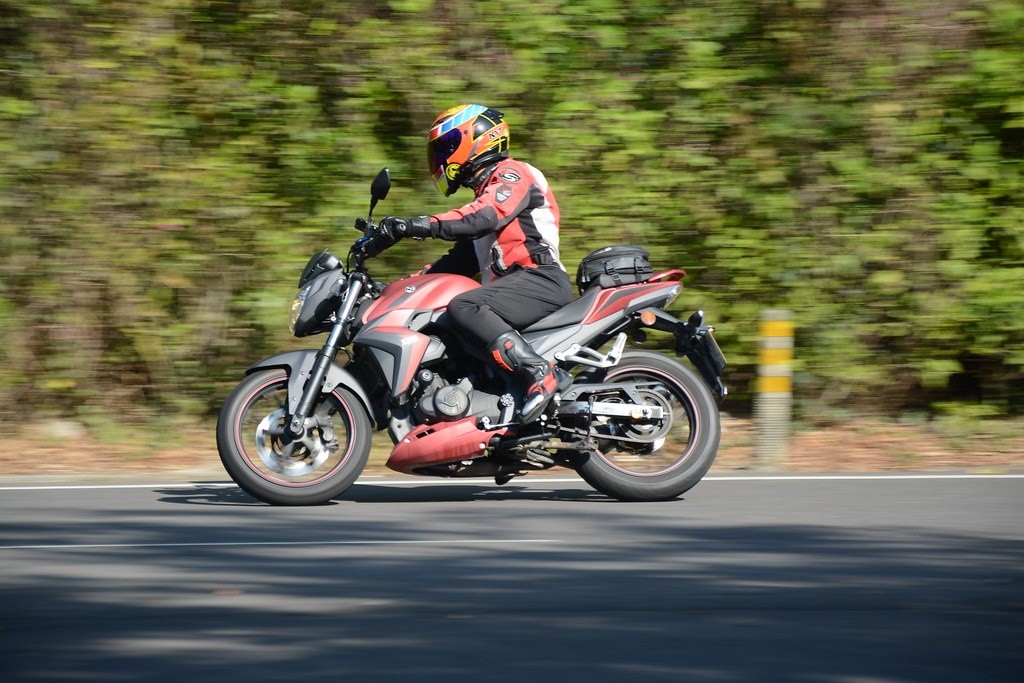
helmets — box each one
[428,102,509,198]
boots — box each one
[485,329,573,426]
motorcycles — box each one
[215,166,731,506]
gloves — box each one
[375,217,430,251]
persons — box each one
[370,104,574,424]
